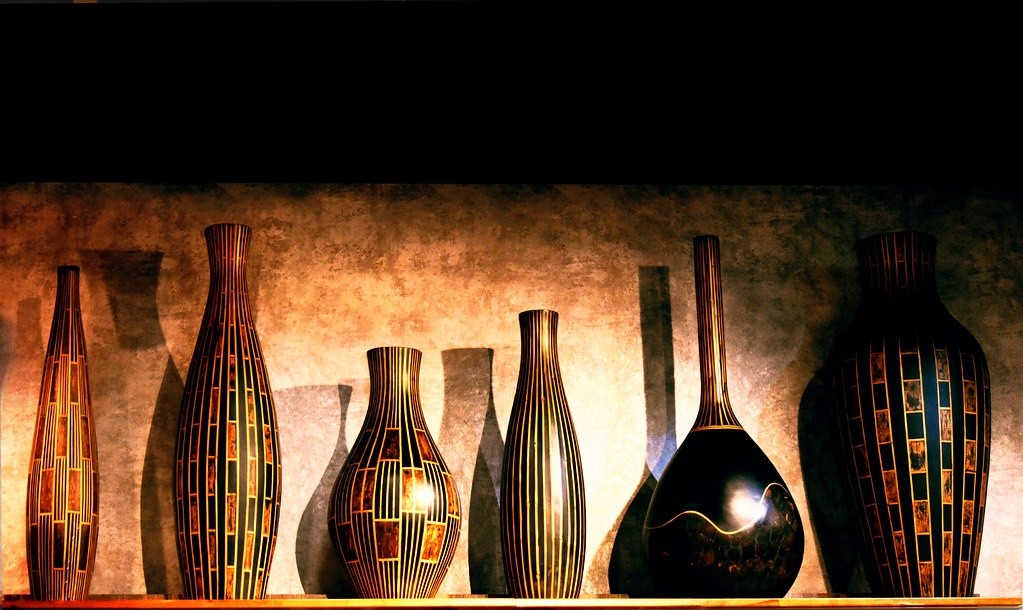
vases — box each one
[498,308,587,598]
[638,228,805,597]
[25,262,105,599]
[837,227,992,596]
[167,220,282,601]
[327,343,464,594]
